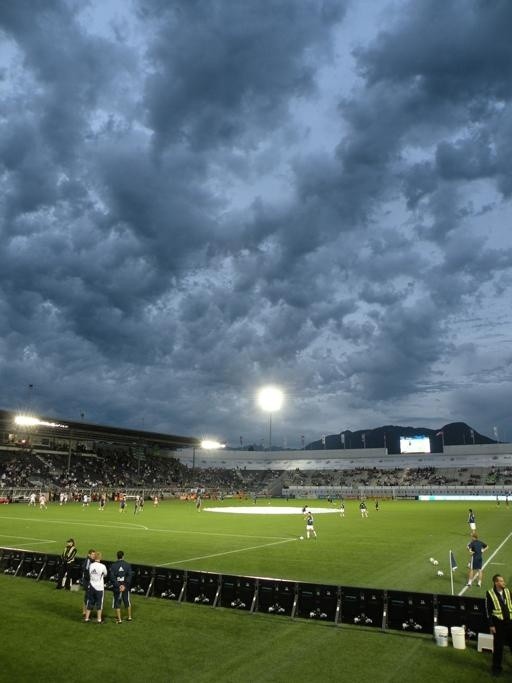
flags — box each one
[450,551,458,573]
[494,428,497,437]
[436,430,443,436]
[283,433,365,448]
[470,430,474,437]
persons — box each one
[485,574,512,677]
[338,498,378,518]
[281,462,512,509]
[56,538,77,591]
[82,549,131,624]
[467,531,489,588]
[467,508,477,536]
[303,511,318,540]
[1,427,280,517]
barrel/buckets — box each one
[435,624,449,647]
[451,627,467,650]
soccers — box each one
[467,563,470,568]
[437,570,444,576]
[300,536,303,539]
[429,558,434,563]
[433,560,438,566]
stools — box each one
[477,633,494,652]
